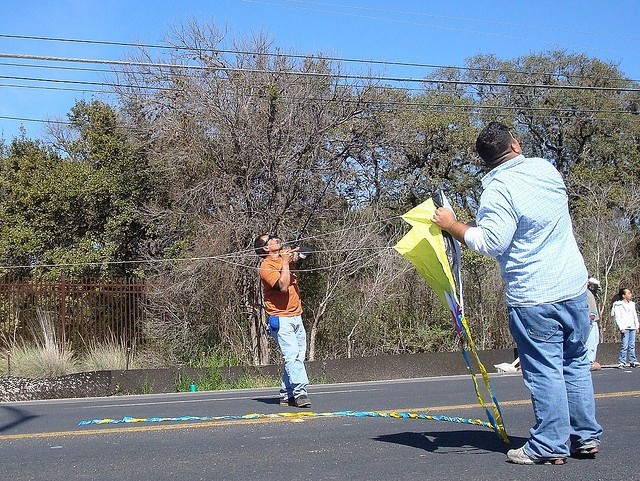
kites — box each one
[76,187,510,443]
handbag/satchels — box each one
[268,316,281,331]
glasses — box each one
[262,235,275,248]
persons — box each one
[430,122,602,465]
[587,278,601,371]
[611,288,640,369]
[254,233,311,407]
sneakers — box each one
[277,397,297,406]
[618,364,626,369]
[507,444,567,466]
[569,444,599,456]
[630,362,640,368]
[294,394,312,409]
